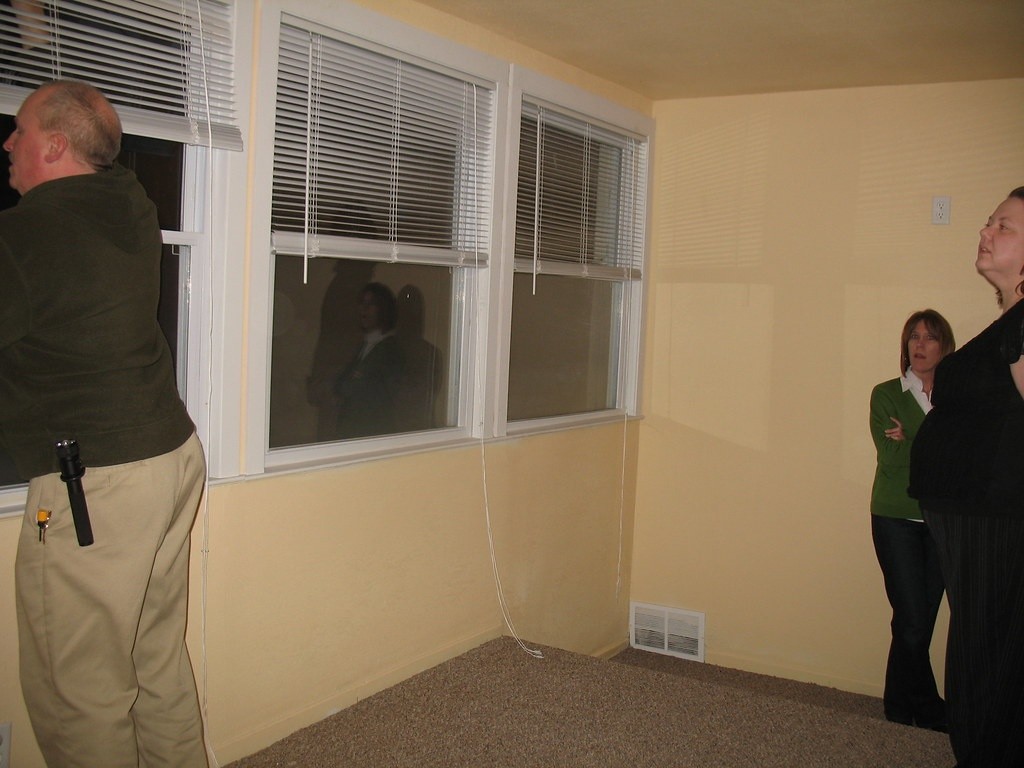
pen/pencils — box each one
[37,511,52,545]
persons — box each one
[906,187,1024,768]
[869,309,955,733]
[0,0,211,768]
